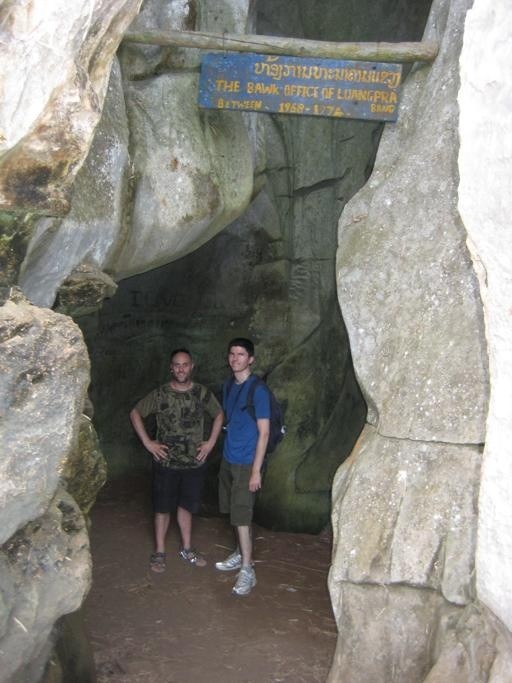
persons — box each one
[128,346,225,574]
[213,337,271,595]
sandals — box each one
[150,549,168,574]
[178,546,207,567]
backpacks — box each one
[225,372,284,453]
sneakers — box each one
[215,544,255,571]
[233,566,257,596]
